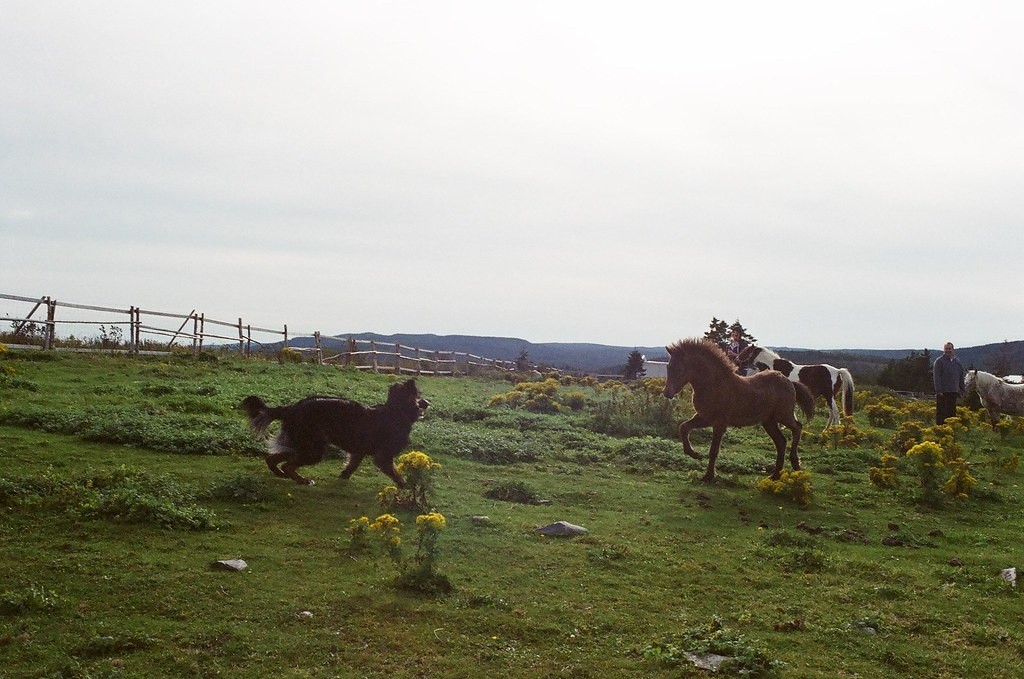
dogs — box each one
[238,379,430,489]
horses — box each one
[733,344,854,435]
[964,368,1024,431]
[662,338,815,483]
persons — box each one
[932,342,964,426]
[727,327,752,376]
